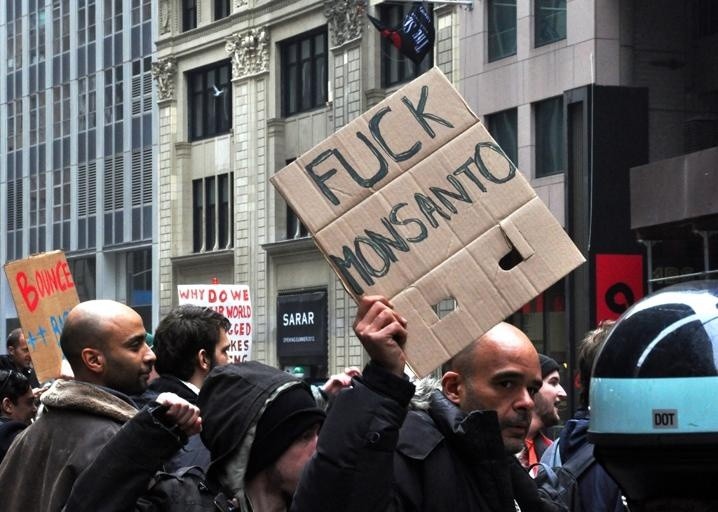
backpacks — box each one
[528,437,595,512]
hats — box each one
[246,385,330,470]
[538,354,561,379]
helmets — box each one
[587,279,718,446]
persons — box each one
[1,279,718,512]
